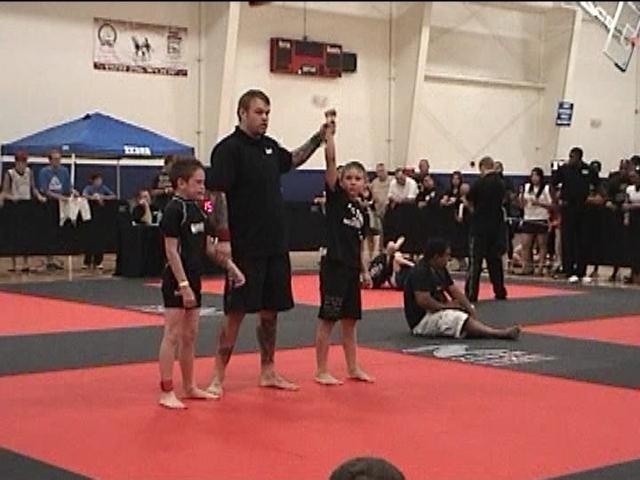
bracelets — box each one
[216,226,232,243]
[178,281,189,287]
[164,186,169,195]
[465,303,475,309]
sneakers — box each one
[568,275,579,283]
[579,276,593,283]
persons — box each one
[209,86,338,394]
[0,152,49,273]
[156,156,246,411]
[132,189,161,227]
[310,103,376,386]
[403,235,521,342]
[152,153,174,224]
[80,173,117,273]
[36,148,74,271]
[312,144,640,292]
